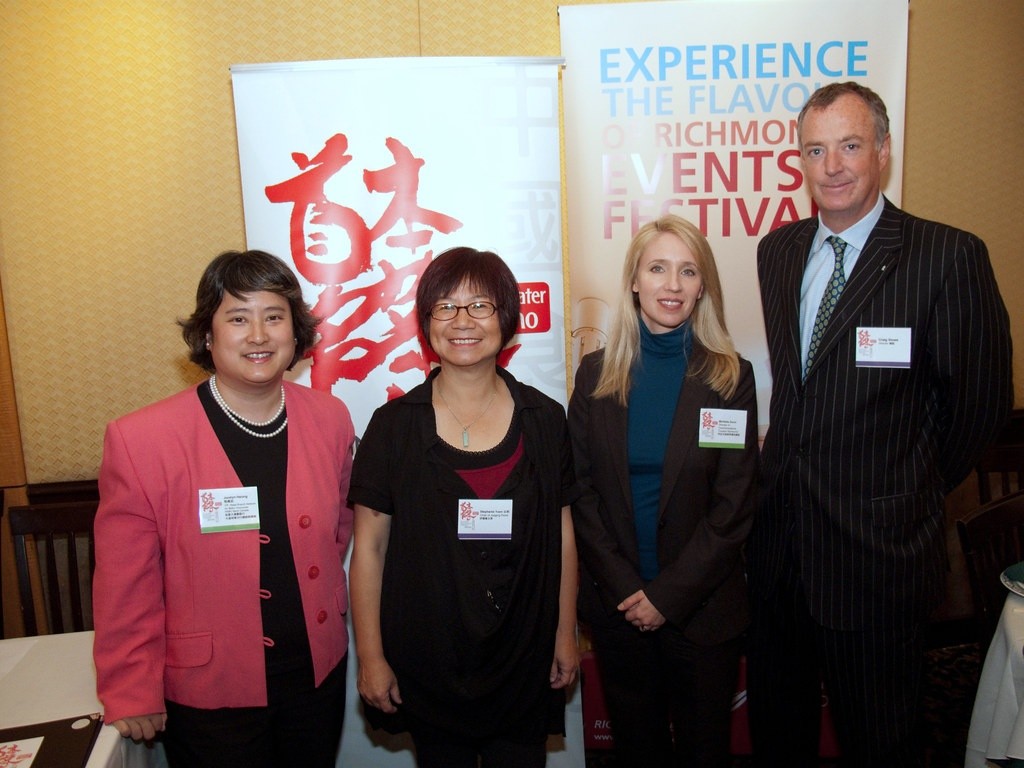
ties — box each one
[803,236,847,385]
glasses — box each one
[430,301,499,321]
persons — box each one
[92,250,354,768]
[346,247,581,768]
[567,214,761,768]
[744,82,1014,768]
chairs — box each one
[8,502,98,636]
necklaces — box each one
[438,385,494,448]
[210,374,287,438]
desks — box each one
[0,626,165,768]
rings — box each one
[642,626,647,632]
